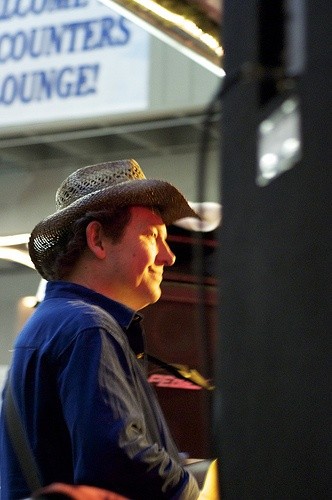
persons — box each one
[0,158,206,500]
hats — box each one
[29,158,204,283]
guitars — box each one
[33,457,213,500]
